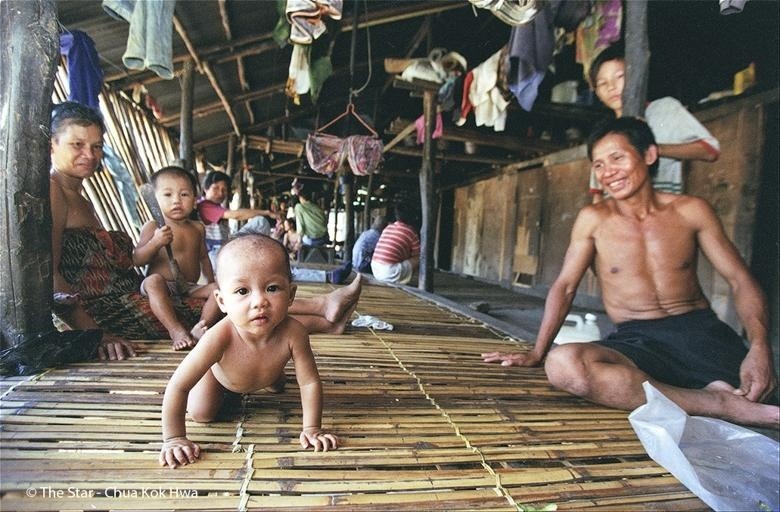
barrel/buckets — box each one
[551,312,601,345]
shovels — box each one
[139,182,192,294]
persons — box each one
[158,232,343,470]
[196,170,352,286]
[48,98,364,362]
[589,45,722,206]
[274,187,330,262]
[132,166,221,352]
[369,201,419,286]
[352,213,388,275]
[480,116,780,432]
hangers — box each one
[314,91,379,139]
